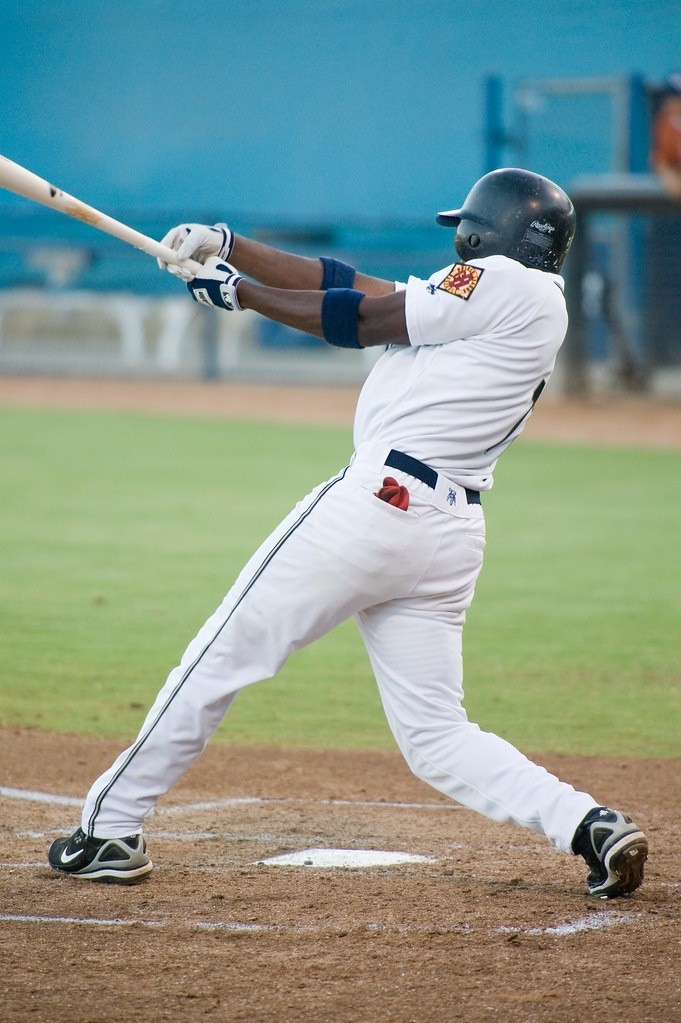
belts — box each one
[384,449,482,505]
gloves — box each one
[186,255,247,311]
[372,477,409,512]
[157,223,235,282]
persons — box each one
[46,168,649,900]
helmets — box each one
[436,167,577,274]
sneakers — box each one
[574,807,648,898]
[48,826,153,884]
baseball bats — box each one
[0,154,204,276]
